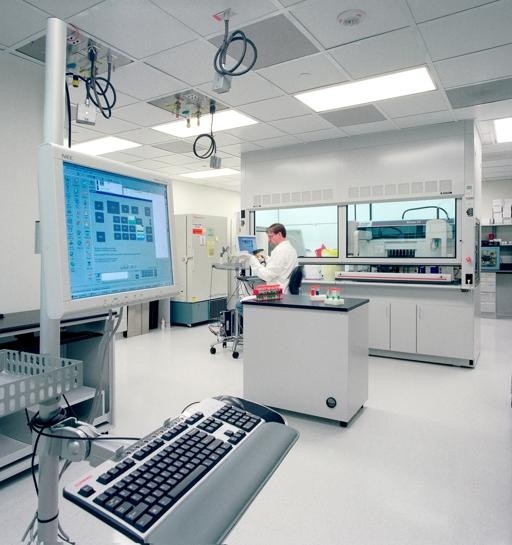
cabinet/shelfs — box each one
[240,292,370,427]
[0,308,117,483]
[170,213,229,328]
[480,223,512,274]
[298,279,482,368]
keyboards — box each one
[62,396,302,544]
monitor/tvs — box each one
[237,235,257,254]
[38,141,180,319]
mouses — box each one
[217,395,247,411]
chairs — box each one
[287,265,304,296]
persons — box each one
[235,223,299,318]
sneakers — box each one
[209,325,220,334]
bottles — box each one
[311,286,315,296]
[325,287,344,305]
[501,240,511,245]
[254,284,283,300]
[316,285,320,295]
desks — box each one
[208,263,251,359]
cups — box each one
[488,233,494,243]
[238,250,251,270]
